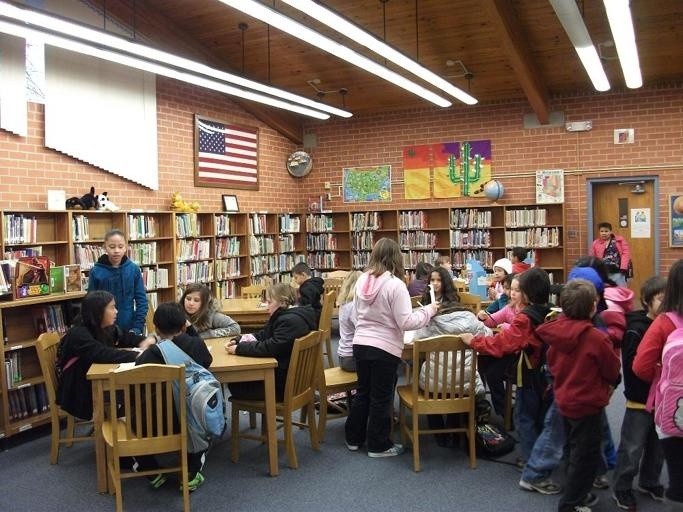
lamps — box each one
[1,0,353,123]
[225,2,477,107]
[547,0,644,90]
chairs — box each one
[240,285,266,298]
[411,295,423,307]
[228,322,326,469]
[35,331,112,465]
[300,327,398,441]
[397,334,477,472]
[319,290,334,368]
[322,276,345,307]
[455,292,481,317]
[101,364,190,512]
[504,377,520,430]
[450,280,466,292]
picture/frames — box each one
[47,190,67,211]
[342,165,392,204]
[536,169,564,204]
[669,193,683,248]
[193,113,259,191]
[223,194,239,212]
[614,129,635,145]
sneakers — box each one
[578,493,599,506]
[150,471,169,488]
[368,444,402,457]
[613,489,635,510]
[561,501,591,511]
[345,439,358,450]
[592,476,610,488]
[520,474,561,494]
[639,481,667,501]
[179,470,203,491]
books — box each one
[177,239,210,261]
[51,264,90,293]
[252,276,278,286]
[452,250,495,269]
[5,351,22,389]
[306,233,337,251]
[127,242,156,265]
[3,213,37,244]
[3,312,9,347]
[506,228,562,249]
[6,245,41,282]
[128,214,155,238]
[399,232,438,248]
[147,291,159,312]
[280,254,305,271]
[216,259,240,281]
[352,231,375,249]
[352,250,371,269]
[307,214,333,233]
[175,214,200,238]
[250,236,275,256]
[402,268,417,284]
[73,213,90,242]
[249,214,267,236]
[250,254,278,276]
[139,266,168,290]
[505,208,548,227]
[452,230,491,248]
[451,209,490,229]
[306,252,341,268]
[178,260,214,284]
[280,215,300,233]
[400,251,439,268]
[9,383,51,422]
[216,237,240,258]
[216,215,231,236]
[74,245,106,265]
[352,211,384,230]
[280,273,292,284]
[399,212,428,230]
[215,282,236,299]
[32,304,62,338]
[279,234,294,253]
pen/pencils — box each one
[416,301,424,307]
[484,309,501,328]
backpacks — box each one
[57,315,89,401]
[646,310,683,437]
[602,234,621,272]
[157,337,227,456]
[471,422,516,458]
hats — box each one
[493,258,512,275]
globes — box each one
[483,179,504,206]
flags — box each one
[197,117,258,186]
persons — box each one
[337,271,364,372]
[434,255,451,276]
[535,277,621,512]
[520,267,608,494]
[589,223,632,288]
[407,262,433,296]
[228,285,316,399]
[420,265,462,309]
[90,228,147,339]
[632,259,683,505]
[507,246,531,274]
[489,258,513,300]
[573,255,633,349]
[346,240,440,458]
[128,300,214,492]
[61,290,152,419]
[294,261,325,311]
[176,282,241,339]
[611,276,668,510]
[479,274,531,328]
[477,272,518,320]
[461,266,554,472]
[413,300,485,448]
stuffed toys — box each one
[67,185,111,210]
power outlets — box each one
[325,182,330,189]
[326,194,331,200]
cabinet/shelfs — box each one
[304,203,566,305]
[0,210,174,438]
[176,208,307,302]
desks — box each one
[322,284,341,295]
[213,299,297,334]
[87,334,278,493]
